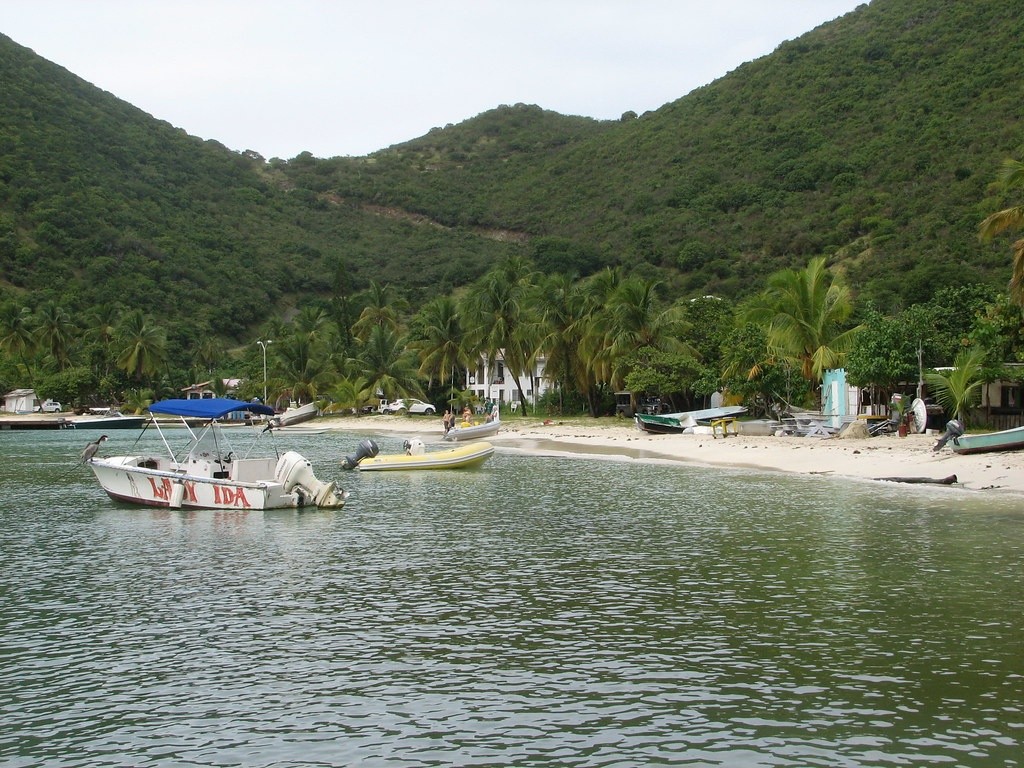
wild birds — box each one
[80,435,111,465]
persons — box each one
[443,397,499,433]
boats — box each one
[932,418,1024,455]
[340,439,496,473]
[633,412,730,435]
[279,400,321,426]
[655,405,749,423]
[86,397,350,512]
[443,419,502,442]
[66,415,147,429]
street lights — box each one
[257,339,272,406]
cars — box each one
[32,401,62,413]
[361,397,436,416]
